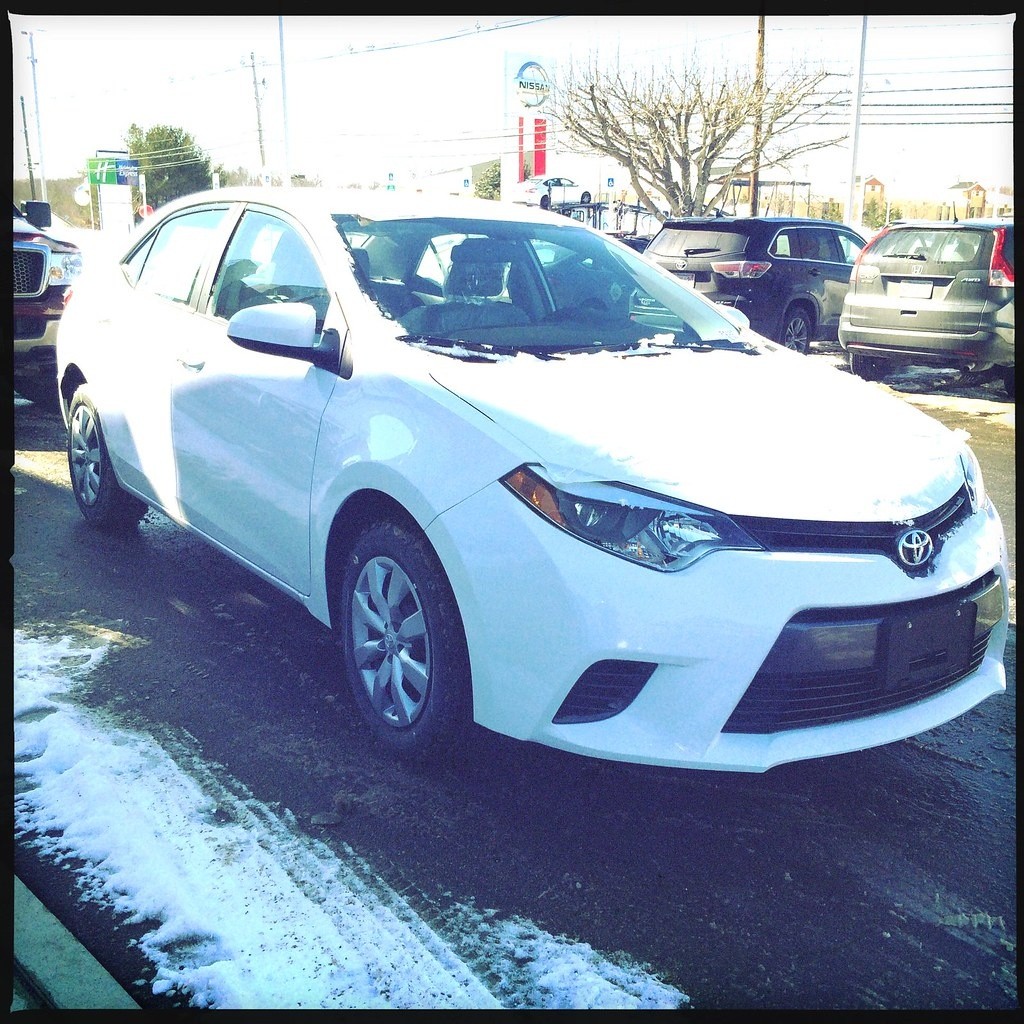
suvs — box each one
[833,217,1016,384]
[627,214,871,355]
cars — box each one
[510,174,594,207]
[538,236,652,320]
[10,200,107,404]
[57,186,1007,774]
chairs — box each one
[401,237,531,336]
[950,243,976,260]
